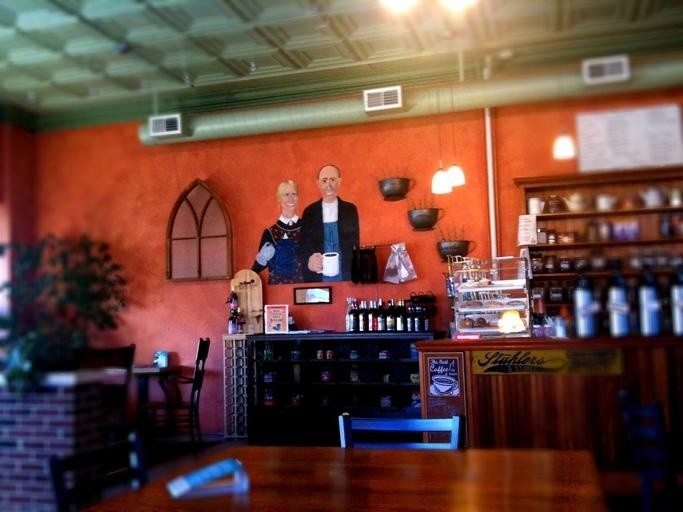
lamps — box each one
[447,82,465,187]
[431,84,452,195]
[552,69,576,160]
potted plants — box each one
[0,233,136,387]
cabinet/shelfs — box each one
[512,165,681,337]
[245,332,434,449]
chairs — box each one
[339,415,460,449]
[141,336,211,454]
[46,441,133,512]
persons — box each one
[300,164,360,284]
[251,180,302,286]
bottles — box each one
[326,350,334,359]
[352,298,358,332]
[560,256,571,272]
[414,302,422,332]
[410,368,420,384]
[537,228,546,245]
[350,350,360,359]
[350,366,358,383]
[364,300,368,331]
[319,369,331,382]
[549,280,563,304]
[548,195,559,213]
[386,298,396,331]
[265,388,271,407]
[410,343,418,361]
[290,350,300,361]
[236,308,244,333]
[396,296,404,331]
[575,255,585,272]
[378,350,389,359]
[545,255,555,272]
[228,309,236,334]
[378,296,385,331]
[264,369,274,384]
[406,302,413,332]
[264,343,270,360]
[547,229,556,243]
[383,370,391,384]
[316,350,324,359]
[359,300,366,331]
[531,253,542,273]
[346,298,356,332]
[368,300,374,332]
[373,300,378,331]
[420,305,428,332]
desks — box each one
[77,446,603,512]
[417,337,683,472]
[131,367,184,452]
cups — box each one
[595,193,618,209]
[315,251,340,277]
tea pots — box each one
[561,193,586,211]
